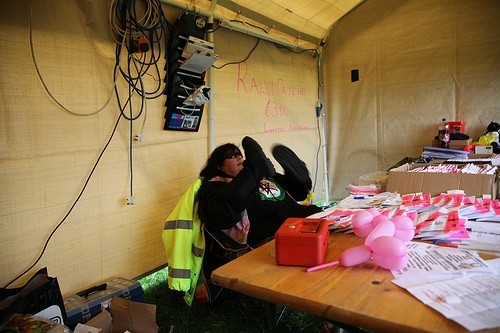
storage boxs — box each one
[275,217,329,267]
[449,138,473,150]
[444,121,465,134]
[386,157,500,200]
[360,171,389,193]
[63,277,158,333]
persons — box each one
[198,136,324,247]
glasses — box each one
[225,151,243,159]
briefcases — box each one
[275,217,328,267]
[64,276,145,332]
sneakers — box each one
[272,144,310,183]
[242,136,277,180]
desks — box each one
[211,194,500,333]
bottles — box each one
[438,118,450,149]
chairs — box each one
[161,179,286,333]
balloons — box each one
[307,207,416,272]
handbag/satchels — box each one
[0,267,69,328]
